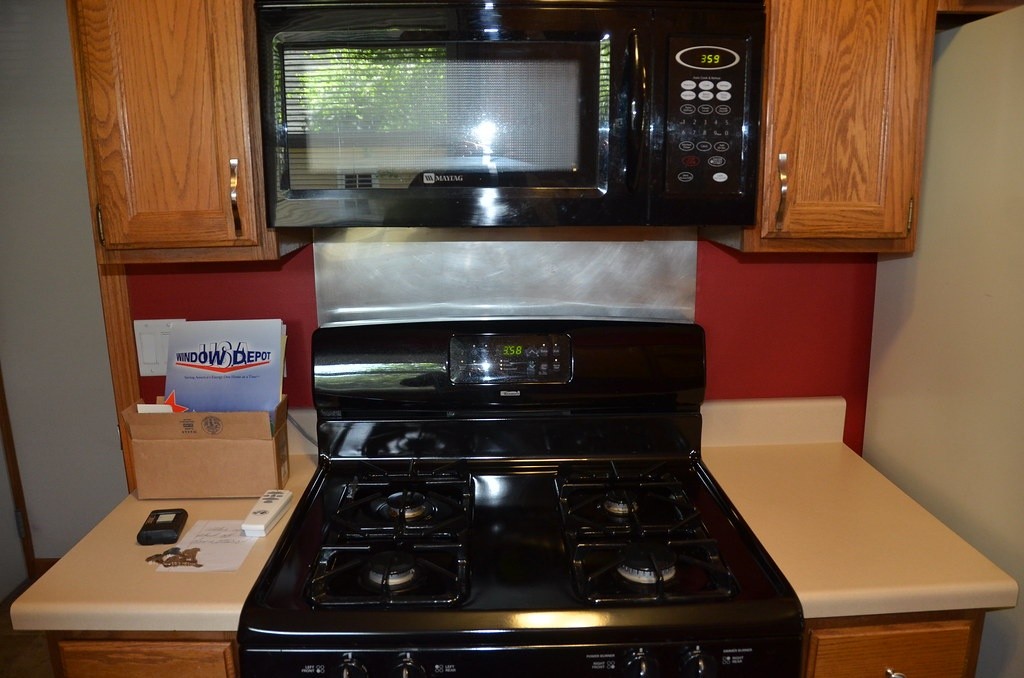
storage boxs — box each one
[122,395,292,499]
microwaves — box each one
[255,0,759,227]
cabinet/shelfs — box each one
[804,622,971,678]
[66,0,314,264]
[699,0,936,255]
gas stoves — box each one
[237,315,805,678]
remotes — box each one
[241,490,293,537]
